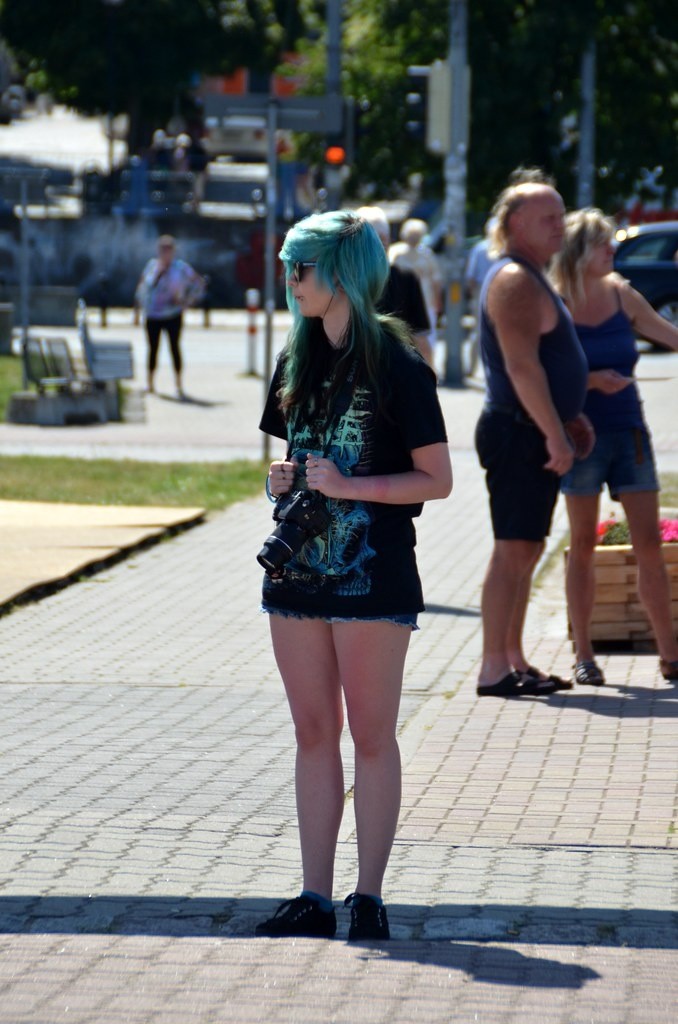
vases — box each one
[565,545,678,640]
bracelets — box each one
[265,474,276,502]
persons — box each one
[477,182,595,696]
[550,207,678,683]
[137,207,513,405]
[255,212,454,945]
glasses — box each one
[292,261,317,283]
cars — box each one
[612,221,678,345]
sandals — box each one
[572,661,605,685]
[659,659,678,680]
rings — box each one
[315,458,319,468]
[280,461,287,480]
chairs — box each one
[22,337,88,398]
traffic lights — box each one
[404,58,448,158]
[327,136,349,170]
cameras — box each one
[257,492,330,572]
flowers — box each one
[597,519,678,545]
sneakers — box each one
[257,896,337,939]
[342,892,390,943]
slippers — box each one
[477,666,573,697]
